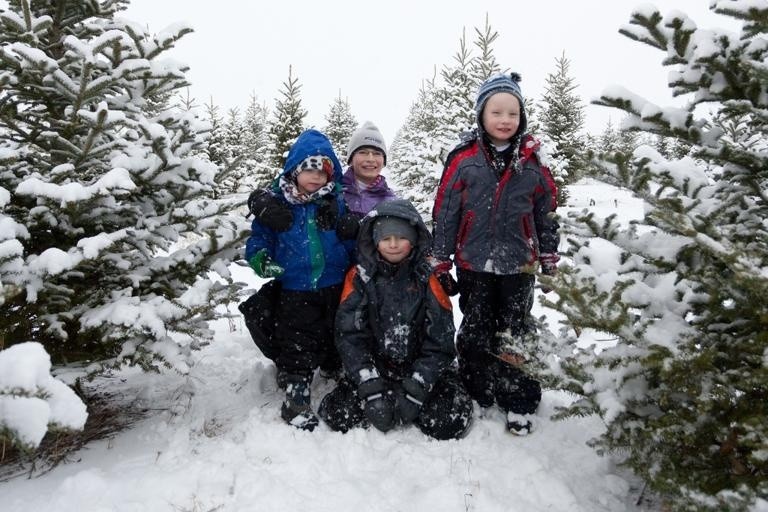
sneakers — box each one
[506,407,538,437]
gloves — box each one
[318,194,363,241]
[433,257,460,296]
[538,251,561,294]
[246,187,294,233]
[344,361,395,433]
[249,245,286,279]
[395,359,441,426]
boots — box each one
[275,366,320,430]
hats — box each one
[345,120,388,169]
[372,216,418,250]
[474,74,527,136]
[290,155,335,183]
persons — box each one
[238,117,401,389]
[244,129,359,432]
[318,200,475,439]
[432,70,561,436]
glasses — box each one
[352,149,386,158]
